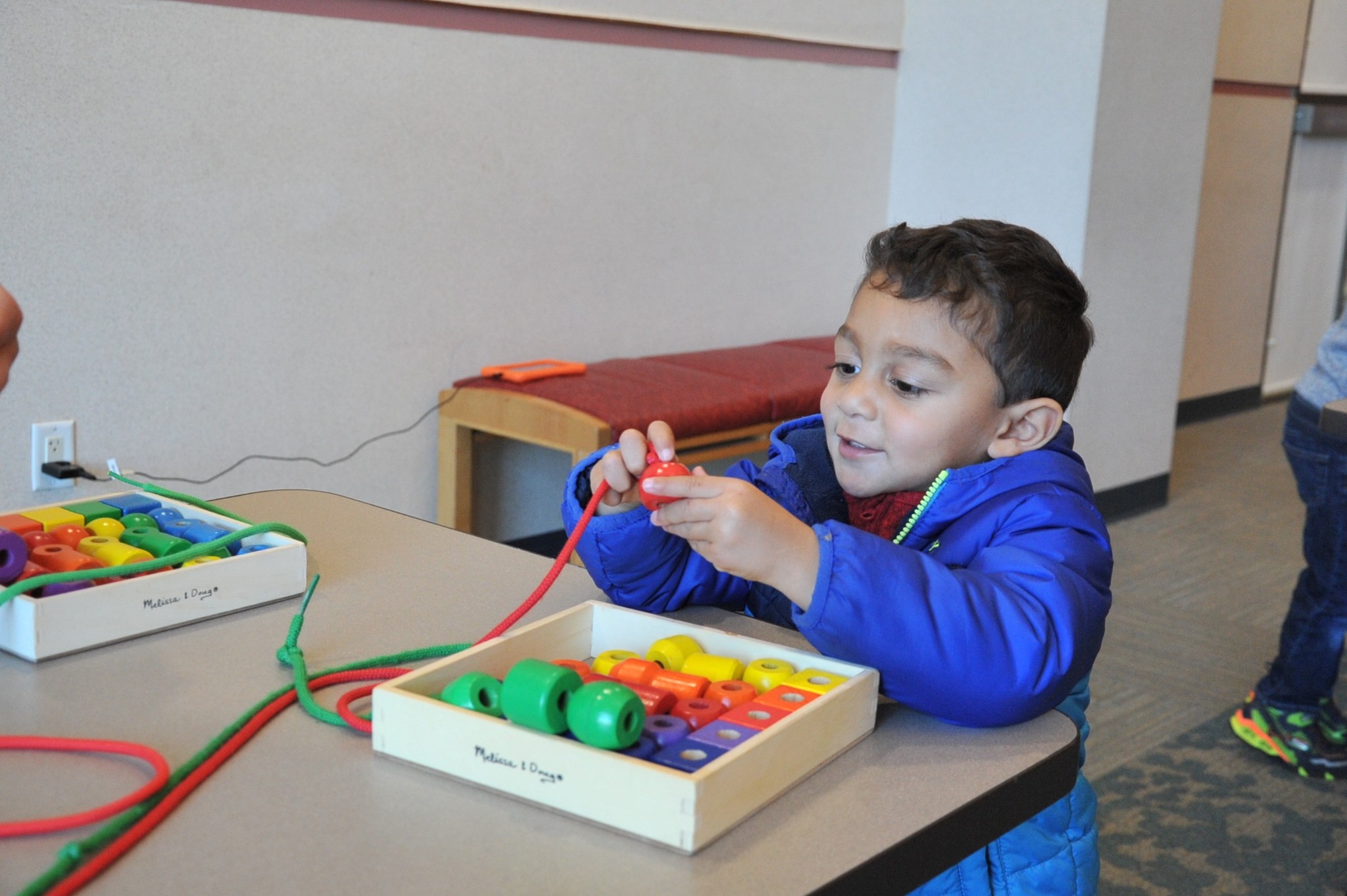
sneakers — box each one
[1310,695,1347,746]
[1226,690,1347,781]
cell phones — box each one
[480,359,582,383]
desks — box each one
[0,488,1082,896]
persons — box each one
[560,218,1113,896]
[1229,306,1347,783]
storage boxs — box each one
[372,598,879,855]
[1,489,308,663]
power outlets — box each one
[30,420,77,492]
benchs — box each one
[438,327,838,581]
